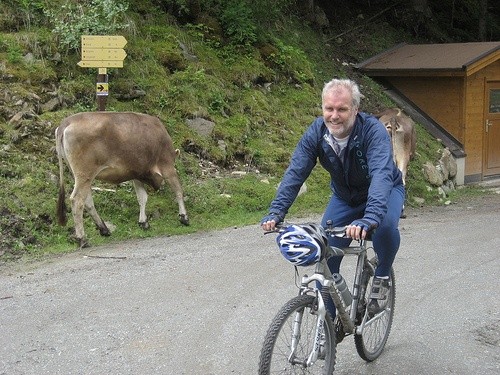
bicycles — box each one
[257,216,407,375]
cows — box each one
[370,108,417,219]
[55,111,190,248]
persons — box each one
[261,79,405,360]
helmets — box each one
[276,222,328,266]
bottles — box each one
[330,273,353,306]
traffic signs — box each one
[77,35,127,75]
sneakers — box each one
[366,275,392,313]
[318,322,328,359]
